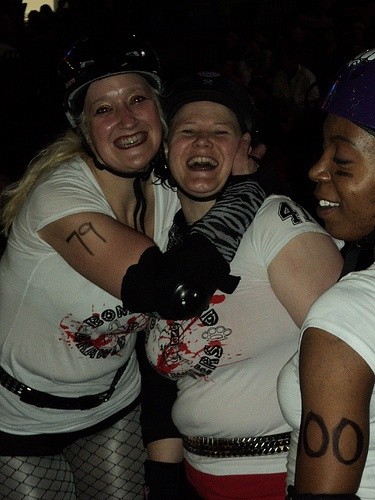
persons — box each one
[0,31,268,500]
[137,0,375,500]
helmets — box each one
[163,59,251,136]
[55,17,167,110]
[308,45,375,138]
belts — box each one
[1,357,139,411]
[179,428,294,459]
